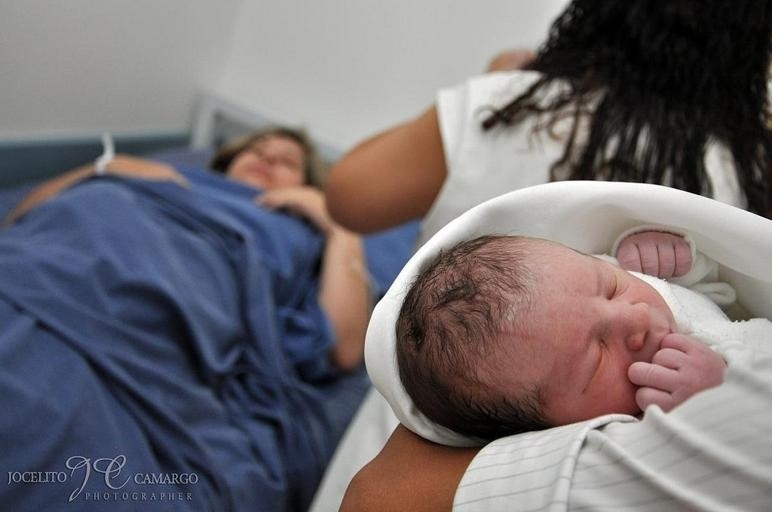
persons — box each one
[334,342,772,512]
[394,220,772,443]
[323,1,772,509]
[1,104,369,512]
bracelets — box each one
[95,135,117,177]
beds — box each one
[0,92,421,511]
